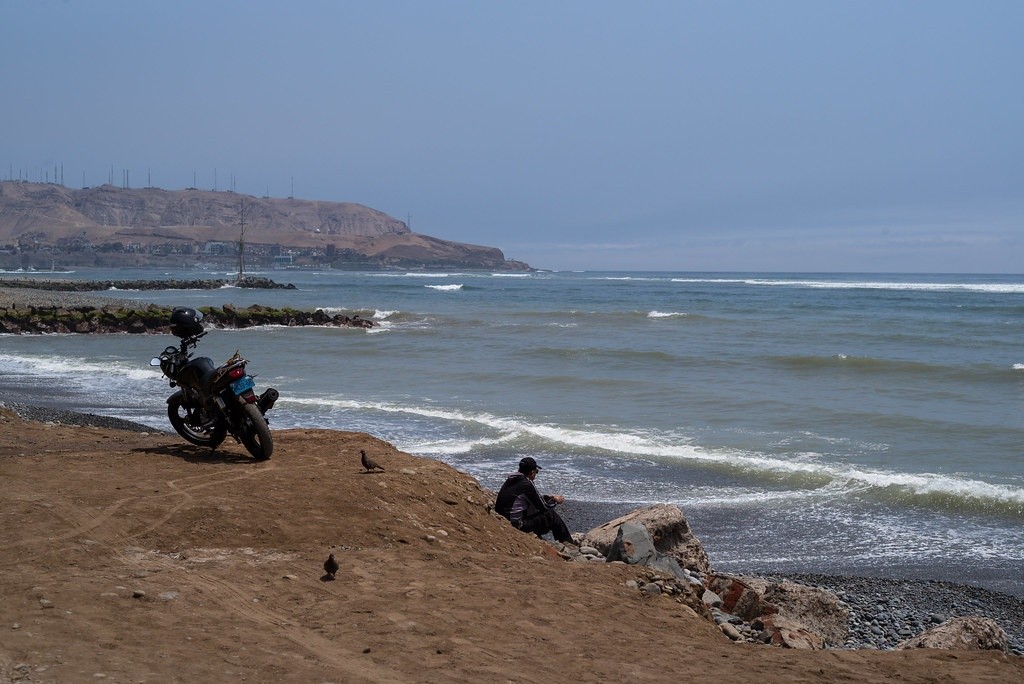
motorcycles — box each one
[150,330,279,461]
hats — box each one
[519,457,542,469]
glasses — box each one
[536,469,539,474]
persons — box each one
[495,456,574,543]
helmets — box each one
[169,305,204,338]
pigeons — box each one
[358,449,386,475]
[323,552,340,579]
